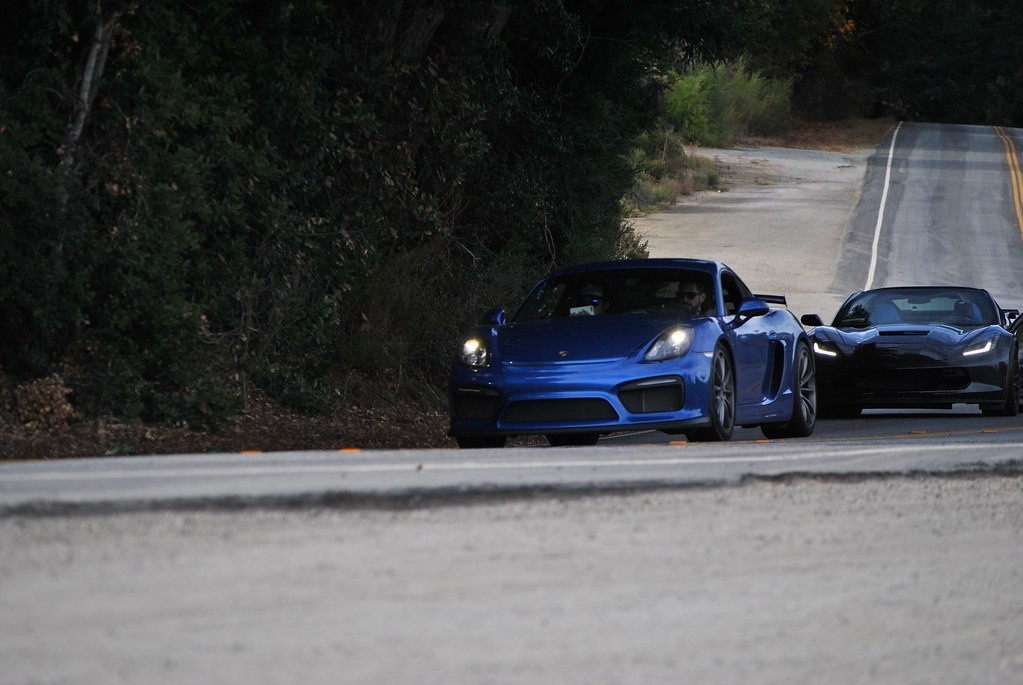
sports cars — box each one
[797,286,1023,420]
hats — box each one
[572,274,609,298]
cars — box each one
[447,258,817,445]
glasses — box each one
[575,296,599,306]
[676,289,705,299]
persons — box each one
[569,283,610,316]
[678,279,706,314]
